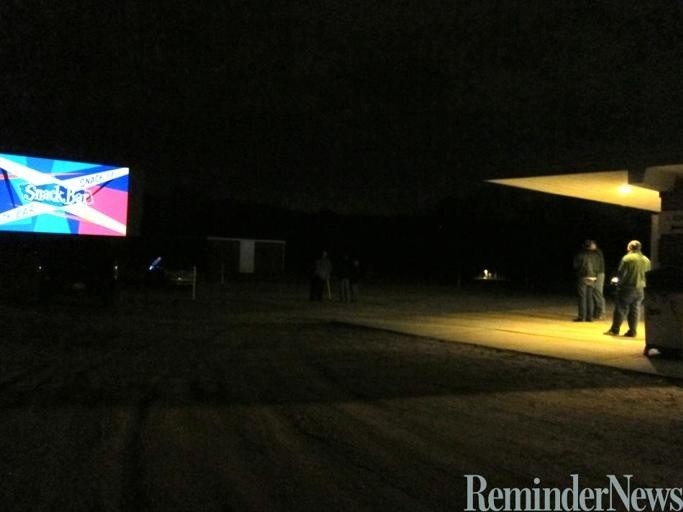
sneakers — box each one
[604,330,636,336]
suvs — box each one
[36,239,125,306]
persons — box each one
[603,239,651,336]
[567,239,601,322]
[309,251,361,304]
[589,240,606,321]
